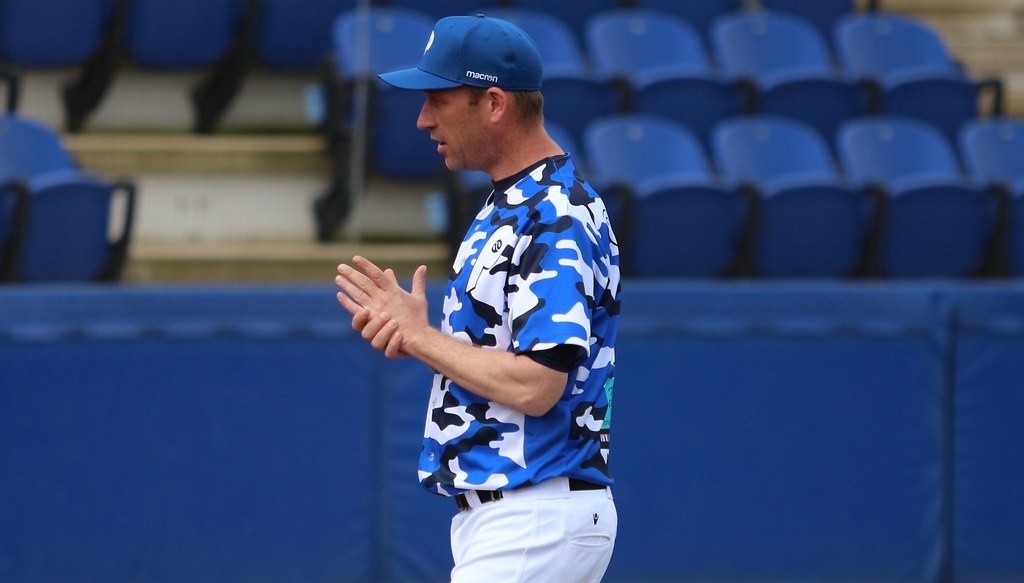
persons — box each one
[334,14,621,583]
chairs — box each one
[0,0,1024,286]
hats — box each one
[375,13,545,93]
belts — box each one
[454,476,608,513]
